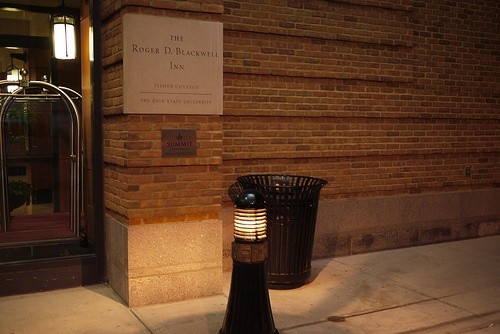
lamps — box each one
[50,7,78,61]
[6,53,26,94]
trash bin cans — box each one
[236,172,327,290]
